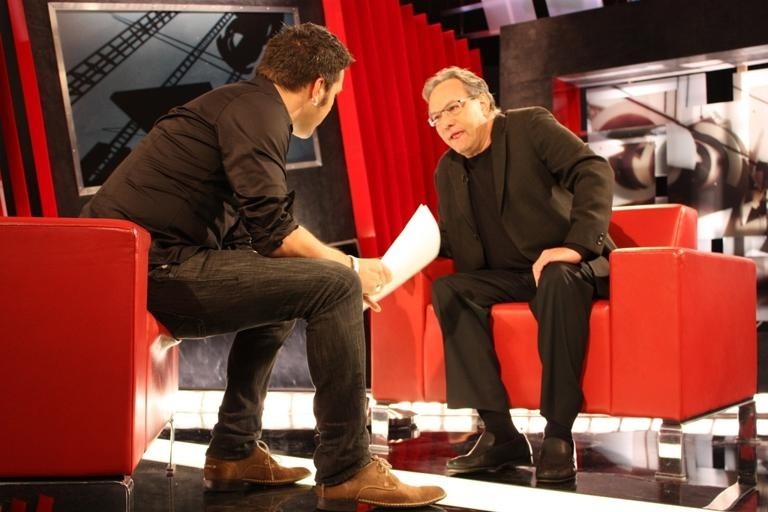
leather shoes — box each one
[534,431,579,485]
[444,426,533,475]
[201,438,312,492]
[314,454,447,511]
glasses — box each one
[427,94,479,128]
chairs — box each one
[0,216,181,512]
[370,205,757,484]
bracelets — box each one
[347,254,360,275]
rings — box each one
[375,284,382,293]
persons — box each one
[418,66,619,485]
[79,23,447,506]
